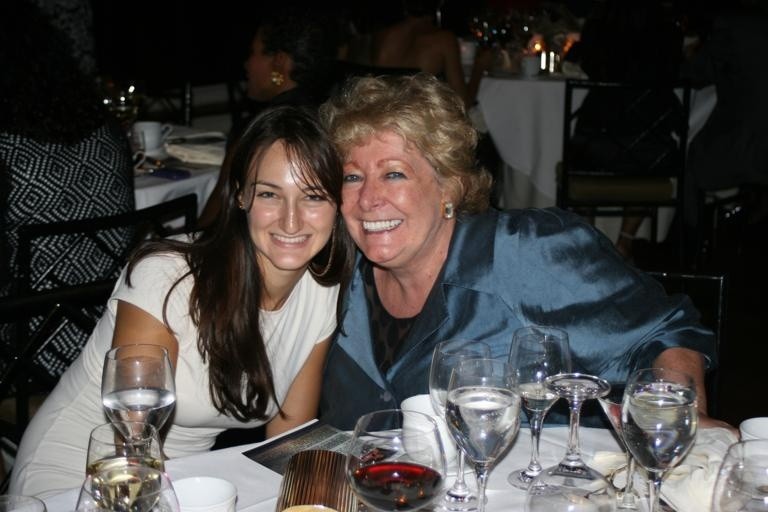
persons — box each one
[4,103,359,511]
[0,49,140,400]
[312,69,745,448]
[185,1,766,251]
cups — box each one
[85,419,166,512]
[710,438,768,512]
[400,394,458,464]
[344,408,448,512]
[74,466,180,511]
[131,121,173,153]
[172,476,238,512]
[738,416,768,441]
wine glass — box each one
[597,382,646,510]
[108,95,138,139]
[429,338,493,512]
[100,343,176,456]
[618,367,700,512]
[444,358,522,511]
[503,326,572,491]
[522,372,618,512]
